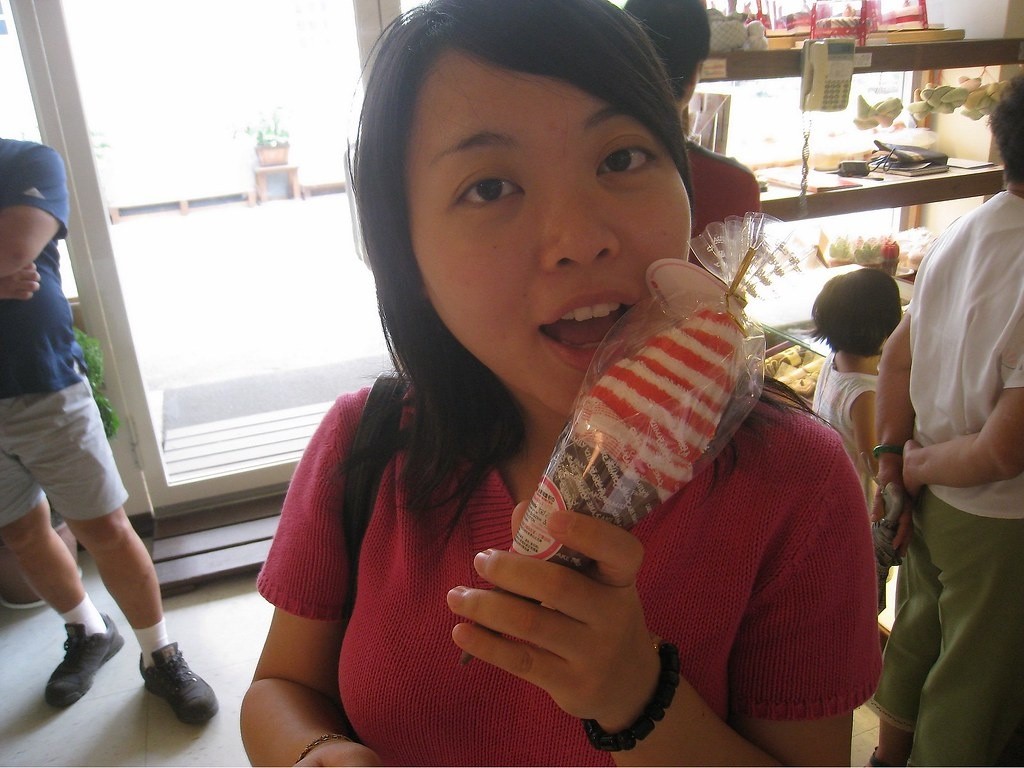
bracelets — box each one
[295,734,353,763]
[580,632,681,752]
[872,444,903,455]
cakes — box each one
[825,234,901,276]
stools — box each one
[255,165,302,202]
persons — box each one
[0,139,218,726]
[811,70,1024,768]
[240,0,882,768]
[622,0,760,279]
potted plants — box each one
[0,329,116,612]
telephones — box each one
[798,38,857,113]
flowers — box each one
[248,118,294,148]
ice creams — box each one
[460,310,744,665]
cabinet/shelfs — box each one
[689,37,1024,222]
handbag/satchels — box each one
[866,140,948,173]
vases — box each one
[256,147,289,165]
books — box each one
[868,159,947,177]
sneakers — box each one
[139,642,219,723]
[46,611,124,704]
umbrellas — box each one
[861,452,903,619]
